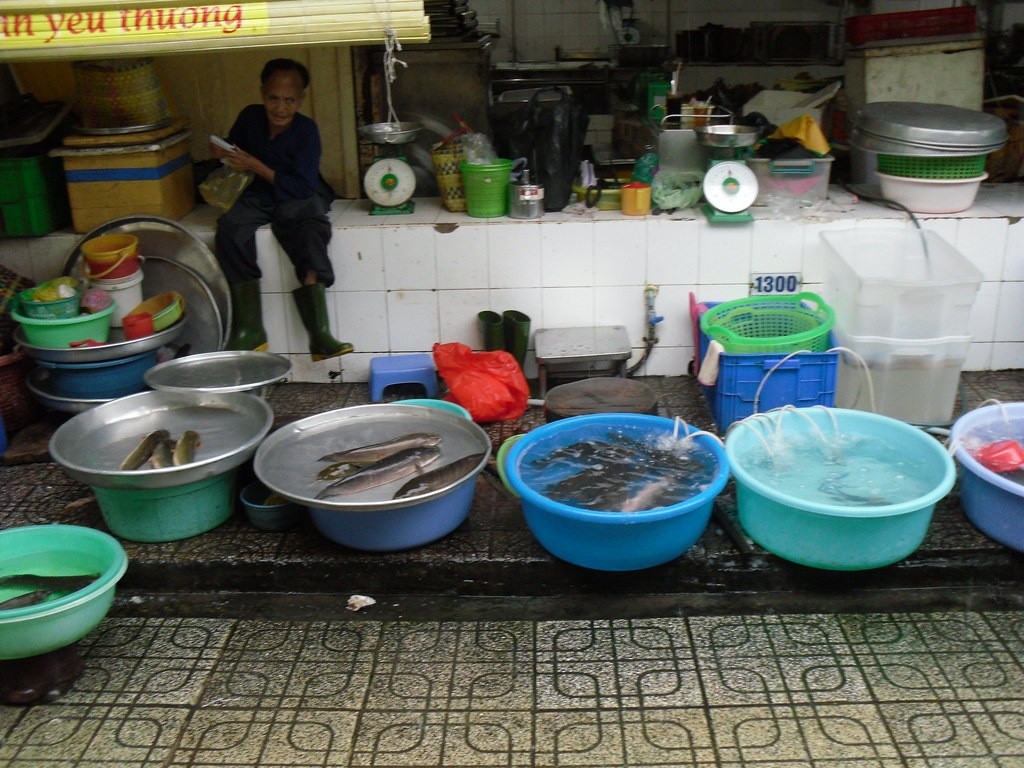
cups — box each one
[507,183,544,219]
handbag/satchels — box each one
[432,342,528,423]
[431,112,475,212]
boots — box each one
[476,311,501,352]
[290,283,354,362]
[501,309,530,373]
[225,279,268,353]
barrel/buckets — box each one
[83,236,143,329]
[19,287,82,319]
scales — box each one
[357,122,426,216]
[615,18,642,45]
[694,125,764,223]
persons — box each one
[207,57,355,361]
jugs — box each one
[622,182,652,216]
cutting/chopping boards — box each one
[544,377,662,422]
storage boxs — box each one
[63,144,197,236]
[749,150,835,207]
[841,1,986,106]
[693,228,983,436]
[0,152,66,238]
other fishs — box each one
[0,574,96,591]
[317,434,441,469]
[0,590,51,611]
[151,440,172,470]
[531,433,703,510]
[172,430,198,468]
[117,429,168,470]
[391,452,487,501]
[817,471,892,506]
[318,462,359,481]
[313,447,440,500]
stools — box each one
[534,323,634,398]
[369,354,438,400]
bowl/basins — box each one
[386,397,472,418]
[91,465,244,543]
[152,298,183,329]
[948,404,1023,558]
[0,525,129,659]
[504,411,728,570]
[304,477,477,552]
[873,169,988,213]
[725,408,955,570]
[29,349,166,397]
[11,298,116,349]
[238,480,300,536]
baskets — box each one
[872,153,985,180]
[699,291,835,355]
